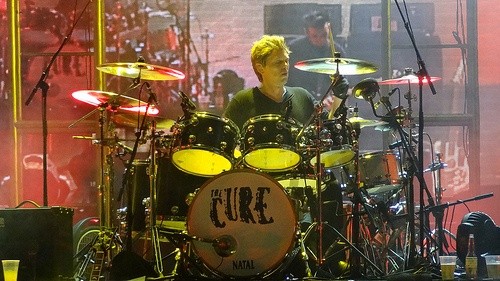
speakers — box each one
[0,207,74,281]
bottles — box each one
[464,234,478,281]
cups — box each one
[485,255,500,281]
[1,260,20,281]
[439,255,457,281]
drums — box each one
[344,151,401,197]
[65,10,112,43]
[20,8,68,45]
[304,119,356,169]
[187,170,298,280]
[272,126,328,194]
[150,155,211,230]
[171,112,242,178]
[239,114,301,171]
[29,42,136,111]
[122,160,170,241]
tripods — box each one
[278,62,456,281]
[68,66,201,281]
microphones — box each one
[389,132,425,149]
[179,90,196,110]
[373,88,397,110]
[146,82,160,106]
[333,95,350,118]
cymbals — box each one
[71,90,159,114]
[294,58,377,75]
[348,117,388,130]
[111,112,177,129]
[379,75,441,84]
[95,63,185,81]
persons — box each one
[284,11,347,119]
[222,34,349,281]
